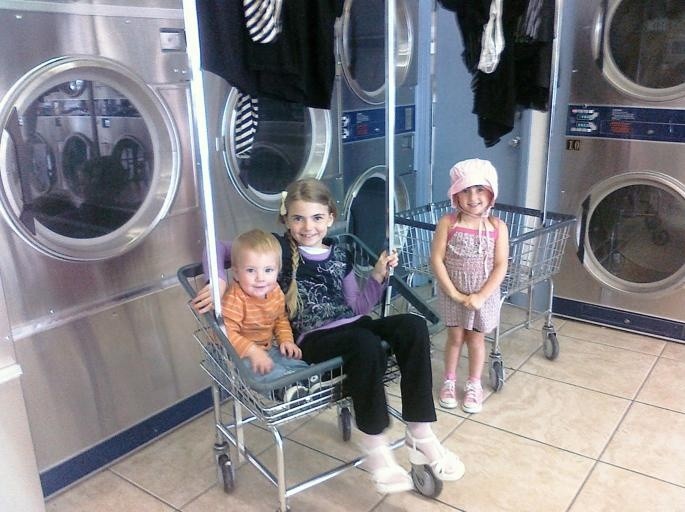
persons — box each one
[217,228,336,410]
[428,156,511,416]
[188,176,468,495]
[65,154,139,242]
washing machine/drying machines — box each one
[327,3,430,313]
[0,2,211,465]
[188,3,331,275]
[538,1,685,349]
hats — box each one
[447,158,498,209]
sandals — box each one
[403,427,466,483]
[357,440,415,497]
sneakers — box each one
[306,376,331,401]
[278,382,309,411]
[438,378,457,409]
[461,380,483,413]
